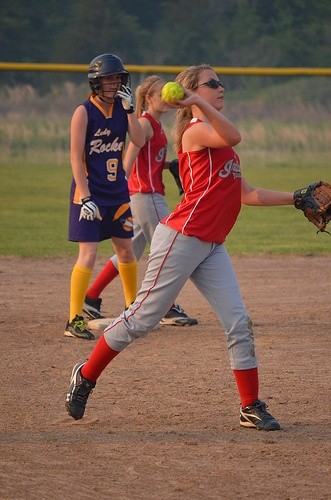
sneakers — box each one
[240,400,281,430]
[158,303,198,325]
[82,296,107,318]
[65,358,96,420]
[64,314,95,340]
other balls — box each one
[161,81,184,105]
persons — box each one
[80,75,199,327]
[64,52,146,340]
[64,65,331,431]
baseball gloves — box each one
[293,181,331,236]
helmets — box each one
[88,54,130,79]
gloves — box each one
[79,196,102,222]
[117,84,135,114]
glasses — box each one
[189,77,224,90]
